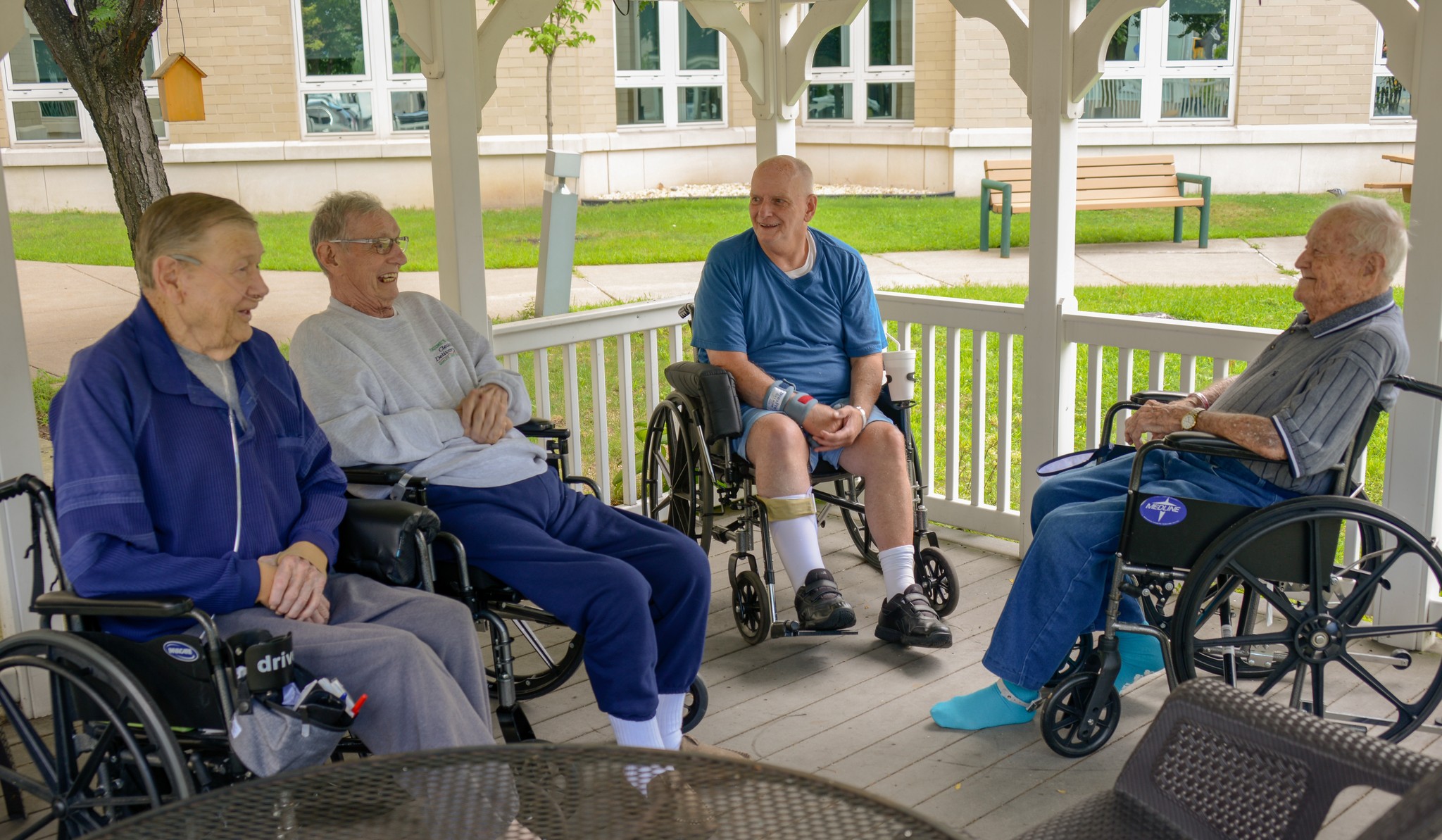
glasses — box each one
[332,236,410,255]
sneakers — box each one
[874,584,952,648]
[795,569,857,631]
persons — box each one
[289,192,764,840]
[48,193,540,840]
[928,197,1411,730]
[691,155,954,650]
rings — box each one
[1140,433,1149,442]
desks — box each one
[1381,152,1416,169]
[69,733,972,840]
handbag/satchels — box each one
[228,631,357,777]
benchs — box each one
[978,154,1214,254]
[1363,180,1411,205]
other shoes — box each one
[622,763,717,835]
[675,734,757,785]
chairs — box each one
[997,671,1442,840]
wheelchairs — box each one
[0,474,571,840]
[1035,373,1441,759]
[341,419,708,825]
[639,302,961,645]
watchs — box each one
[1179,407,1205,432]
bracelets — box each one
[783,392,818,428]
[855,405,867,430]
[1189,391,1211,408]
[762,379,797,412]
